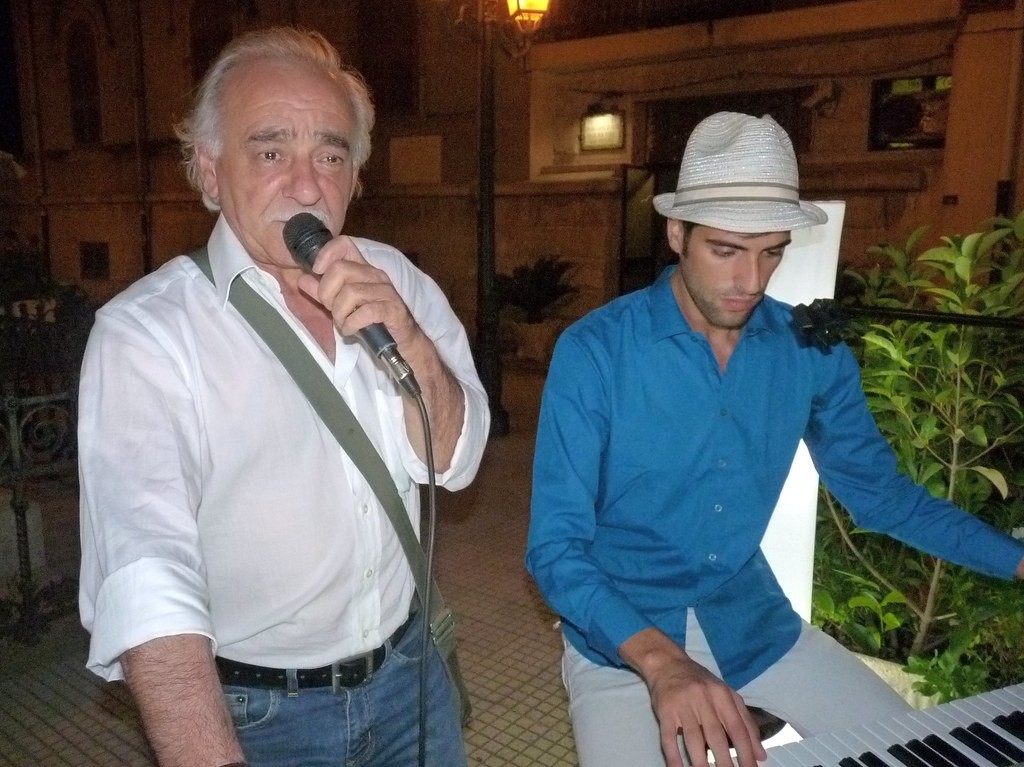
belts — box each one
[214,612,417,689]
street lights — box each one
[475,1,551,437]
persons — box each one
[77,20,491,767]
[524,110,1024,767]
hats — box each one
[652,111,828,233]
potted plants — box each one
[493,256,581,360]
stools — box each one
[704,704,786,746]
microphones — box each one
[282,213,422,398]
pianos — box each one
[716,682,1024,765]
